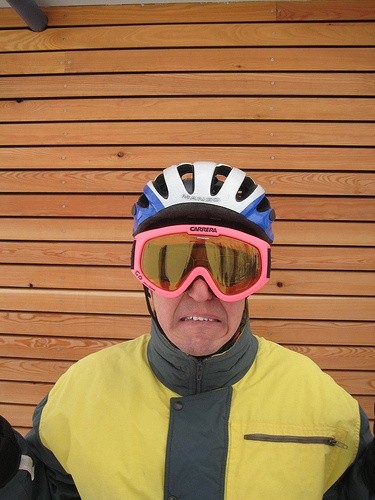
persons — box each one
[1,160,375,500]
[158,241,214,289]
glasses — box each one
[129,225,270,301]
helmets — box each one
[133,161,276,245]
